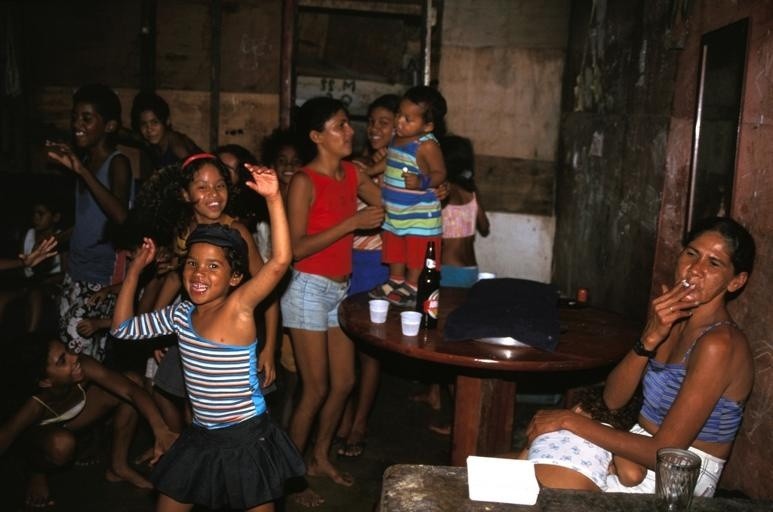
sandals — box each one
[386,282,418,307]
[368,279,402,299]
[106,462,154,490]
[25,470,58,510]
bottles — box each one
[419,241,439,329]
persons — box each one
[112,162,292,512]
[0,331,180,508]
[131,90,205,242]
[149,152,279,463]
[518,384,648,491]
[280,95,450,509]
[524,216,757,497]
[75,216,171,388]
[273,136,305,203]
[364,84,447,305]
[215,143,274,265]
[329,94,401,460]
[44,82,128,363]
[21,198,72,306]
[0,236,58,270]
[441,182,490,266]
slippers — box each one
[336,436,346,455]
[308,461,352,487]
[294,490,326,508]
[338,438,368,455]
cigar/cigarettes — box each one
[680,280,690,287]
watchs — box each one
[634,338,656,358]
[418,174,430,191]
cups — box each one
[655,448,701,512]
[369,300,389,324]
[401,311,422,336]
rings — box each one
[668,306,674,312]
